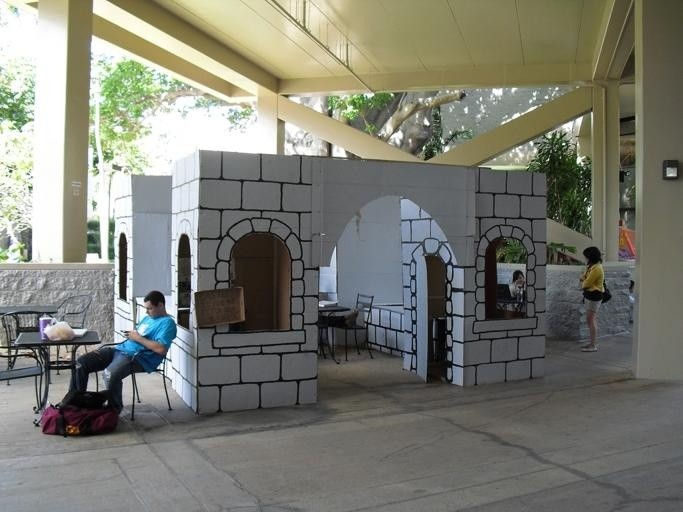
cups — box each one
[39,317,52,340]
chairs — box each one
[0,294,173,421]
[316,292,374,365]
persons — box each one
[508,270,526,297]
[69,291,177,414]
[579,247,605,352]
[629,280,635,323]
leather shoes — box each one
[580,342,600,352]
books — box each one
[319,301,337,307]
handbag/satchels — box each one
[601,282,612,304]
[41,390,123,436]
